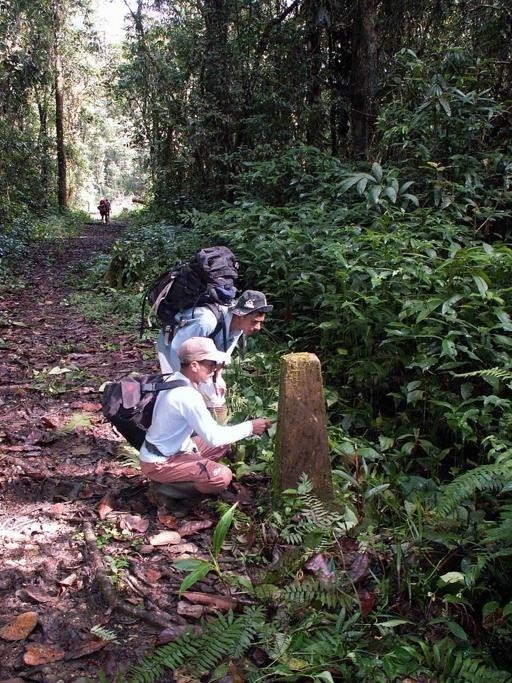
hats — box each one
[176,337,229,363]
[228,290,273,316]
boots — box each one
[147,481,201,512]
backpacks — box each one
[148,246,239,340]
[103,372,188,451]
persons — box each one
[97,198,111,224]
[156,290,274,408]
[138,337,273,518]
